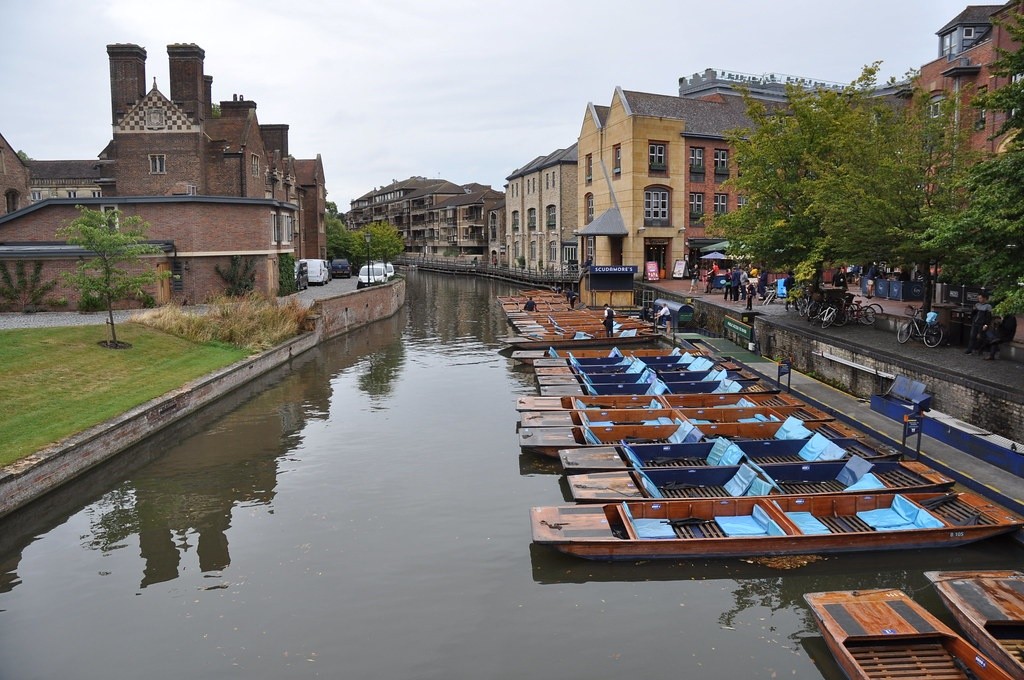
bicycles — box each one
[896,305,944,348]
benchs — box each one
[873,314,918,338]
[998,340,1024,364]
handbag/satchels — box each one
[726,282,731,286]
[706,277,713,283]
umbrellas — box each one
[700,252,733,260]
[700,241,754,252]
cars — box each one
[331,259,354,278]
[370,263,394,278]
[292,258,333,290]
[359,266,388,288]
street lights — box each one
[364,229,373,288]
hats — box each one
[788,271,795,275]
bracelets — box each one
[984,325,988,328]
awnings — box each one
[575,207,628,235]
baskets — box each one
[905,307,914,316]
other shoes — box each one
[964,350,972,354]
[745,308,749,309]
[705,291,709,293]
[795,308,799,312]
[785,308,788,312]
[990,340,999,345]
[749,308,752,310]
[984,356,994,360]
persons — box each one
[566,289,576,310]
[688,261,883,311]
[639,303,671,334]
[604,304,616,337]
[963,294,1017,361]
[523,297,537,312]
[492,256,498,268]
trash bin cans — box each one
[962,311,972,347]
[949,307,969,347]
[824,286,846,324]
[930,303,957,346]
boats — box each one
[528,490,1024,561]
[564,459,956,505]
[557,436,904,473]
[923,568,1024,680]
[495,281,886,460]
[803,587,1017,680]
